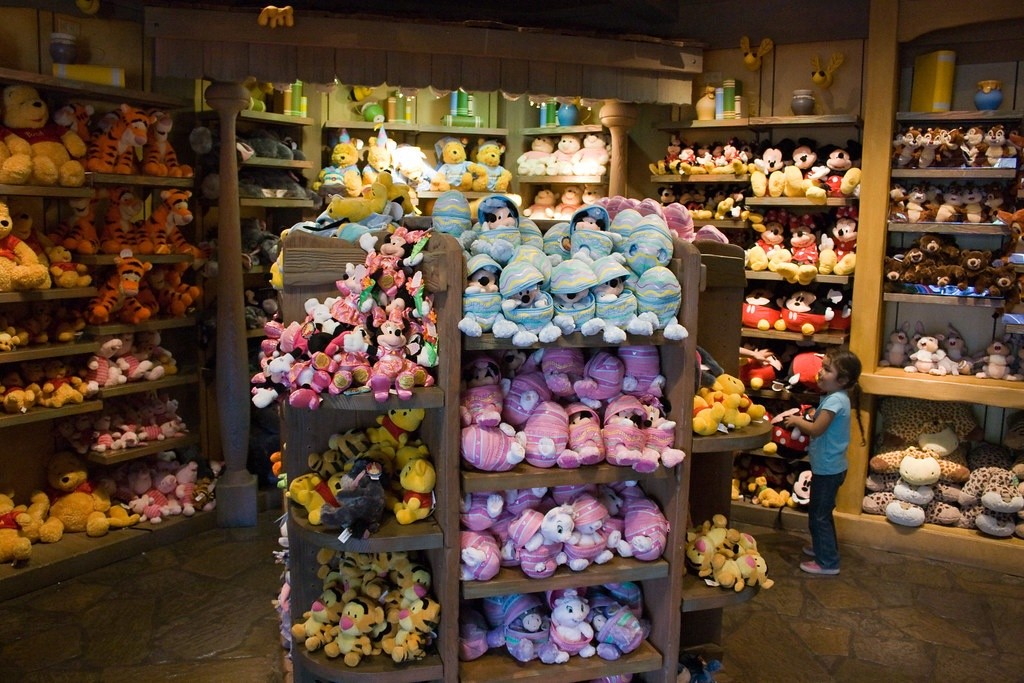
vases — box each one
[791,89,816,115]
[558,103,580,126]
[360,102,384,122]
[695,85,717,120]
[973,79,1003,110]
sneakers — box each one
[803,543,818,556]
[800,560,841,574]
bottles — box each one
[974,80,1003,111]
[558,102,579,126]
[361,102,385,122]
[696,86,716,121]
[49,32,78,65]
[790,90,815,116]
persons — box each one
[784,347,866,575]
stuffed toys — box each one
[0,82,1024,683]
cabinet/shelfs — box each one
[321,121,509,198]
[0,67,198,465]
[515,125,612,228]
[274,218,774,683]
[195,110,314,379]
[883,110,1023,332]
[651,115,865,460]
